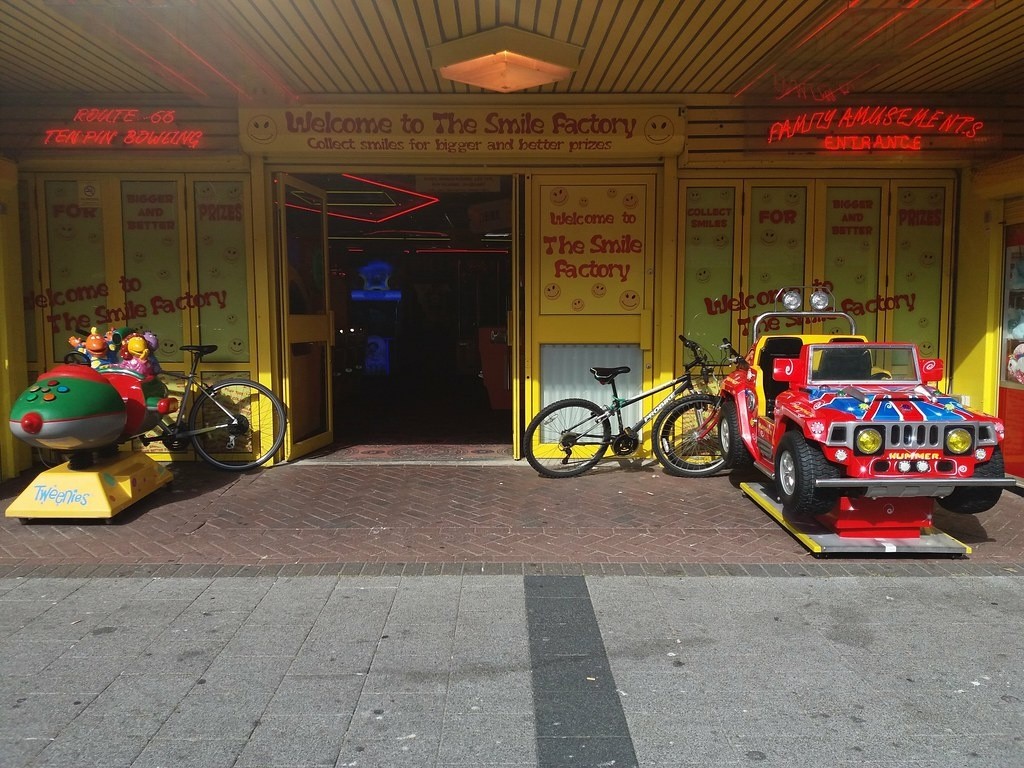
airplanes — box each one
[8,325,179,452]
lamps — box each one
[427,25,583,92]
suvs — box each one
[720,333,1017,520]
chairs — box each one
[760,336,804,414]
[819,338,873,380]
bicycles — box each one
[652,336,752,477]
[31,344,287,474]
[522,334,739,478]
